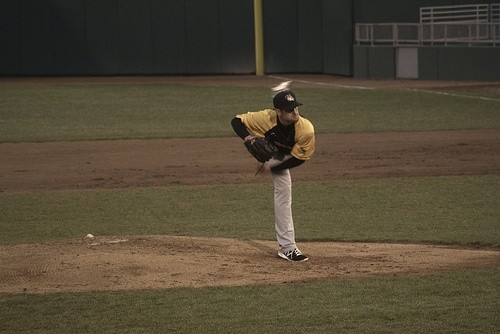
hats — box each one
[273,90,303,112]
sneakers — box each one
[277,248,309,263]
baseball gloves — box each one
[242,139,285,176]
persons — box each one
[231,81,315,263]
